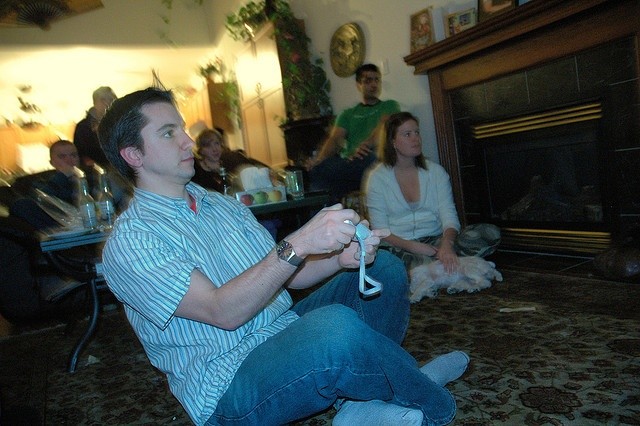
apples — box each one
[269,189,282,203]
[254,190,268,204]
[240,192,254,205]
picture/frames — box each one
[408,5,436,56]
[441,7,478,39]
[475,0,520,24]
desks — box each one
[40,190,339,373]
[402,1,640,274]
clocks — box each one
[330,20,368,80]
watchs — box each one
[274,238,304,270]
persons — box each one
[74,87,122,204]
[307,65,401,187]
[194,130,241,197]
[96,70,469,426]
[364,111,501,282]
[32,139,92,225]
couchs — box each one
[0,169,113,323]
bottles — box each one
[79,177,97,231]
[99,174,117,230]
[218,166,233,197]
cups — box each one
[287,170,305,200]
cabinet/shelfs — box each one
[233,17,318,176]
